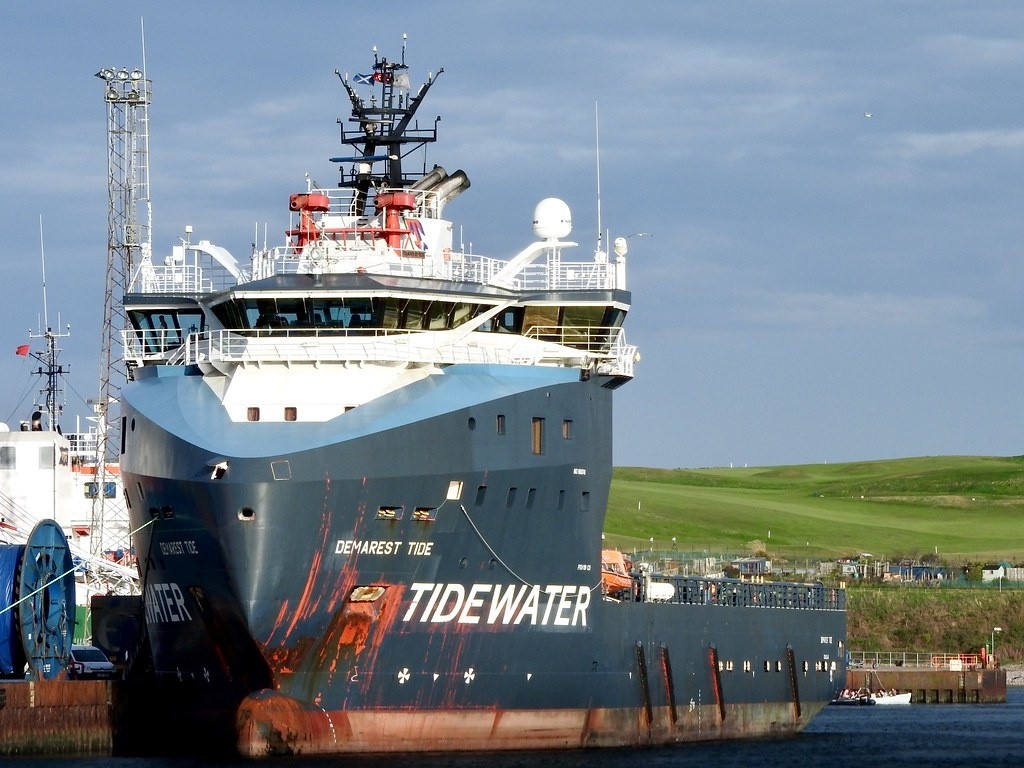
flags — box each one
[393,74,410,88]
[354,73,374,86]
[373,72,390,82]
[16,345,29,355]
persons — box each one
[68,657,79,676]
[839,688,896,700]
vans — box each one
[68,646,116,682]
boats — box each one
[830,691,912,705]
[0,209,142,643]
[600,549,632,596]
[114,35,851,768]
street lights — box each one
[992,627,1002,667]
